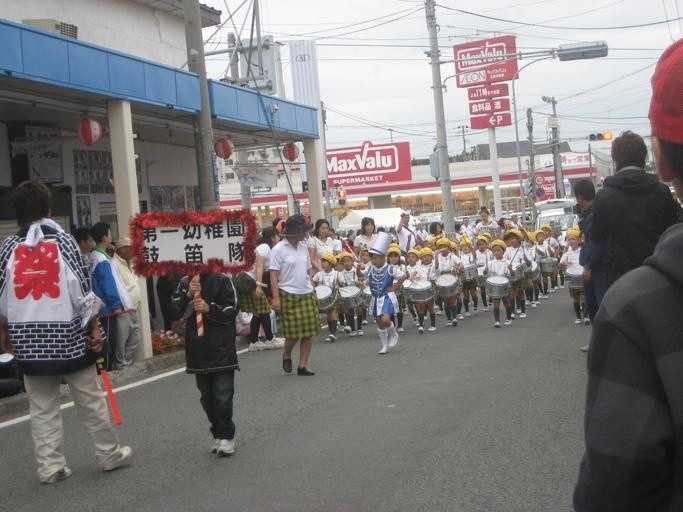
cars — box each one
[416,198,533,225]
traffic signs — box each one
[452,33,520,89]
[469,112,511,130]
[299,178,326,192]
[468,98,510,116]
[467,82,509,101]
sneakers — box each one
[102,442,133,471]
[43,467,72,483]
[248,336,285,352]
[209,436,221,453]
[398,327,403,332]
[217,438,236,455]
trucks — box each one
[532,198,578,234]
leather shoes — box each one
[412,317,436,332]
[511,308,527,320]
[504,319,512,326]
[436,305,489,319]
[282,353,293,374]
[584,317,589,324]
[297,366,315,375]
[574,318,581,325]
[446,320,452,326]
[526,284,565,308]
[337,320,369,336]
[328,333,337,342]
[452,317,458,326]
[494,322,500,328]
[325,337,331,342]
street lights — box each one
[523,157,530,175]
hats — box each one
[491,239,506,251]
[649,39,682,144]
[279,214,314,239]
[369,231,393,257]
[407,235,458,258]
[459,236,472,247]
[505,229,524,242]
[321,250,353,265]
[528,224,552,241]
[388,242,402,256]
[565,227,580,239]
[478,232,492,243]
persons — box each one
[166,267,240,456]
[574,132,683,351]
[268,214,322,376]
[1,180,133,484]
[71,222,142,373]
[572,38,683,512]
[233,208,590,353]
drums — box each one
[315,258,583,312]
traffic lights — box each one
[588,132,612,141]
[338,190,345,206]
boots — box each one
[386,322,399,348]
[376,327,388,354]
